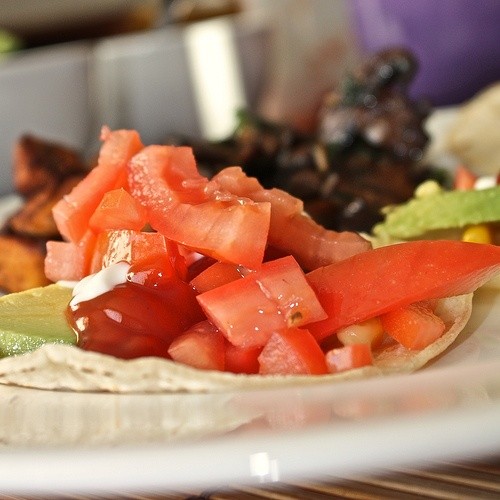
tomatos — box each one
[42,124,500,376]
